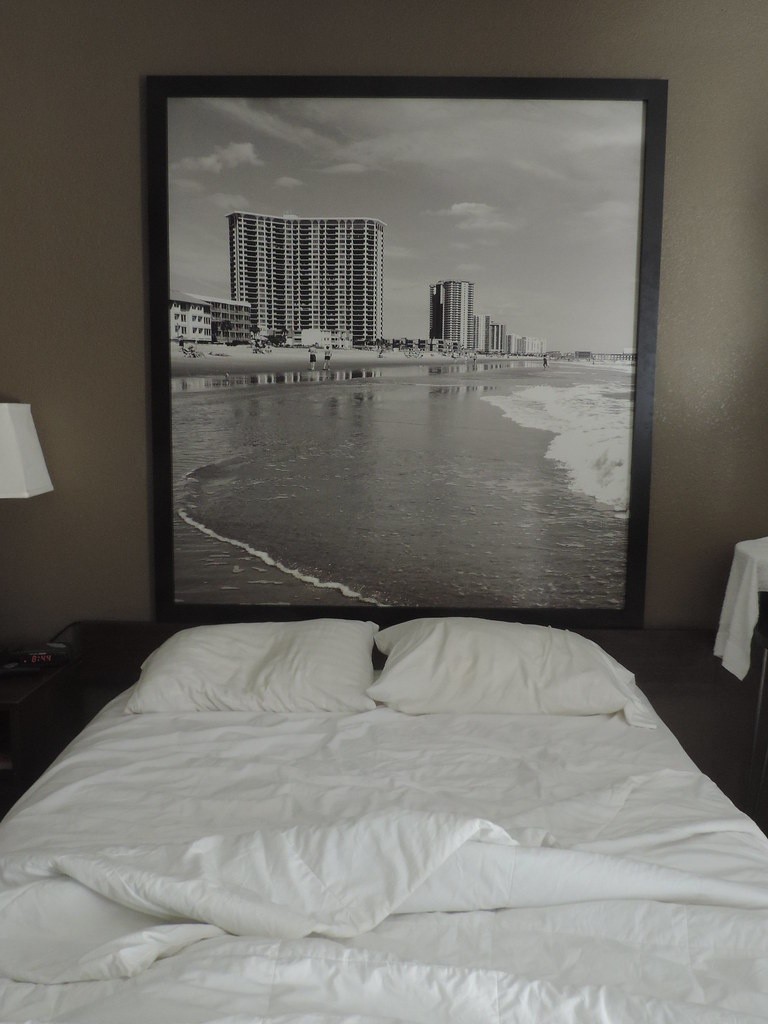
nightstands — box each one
[0,659,79,824]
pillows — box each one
[123,618,380,716]
[366,617,658,730]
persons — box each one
[253,340,272,354]
[177,334,184,351]
[473,353,476,361]
[323,345,332,371]
[543,355,547,368]
[308,343,317,370]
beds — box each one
[0,670,768,1024]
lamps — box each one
[0,403,54,500]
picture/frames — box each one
[139,73,668,634]
